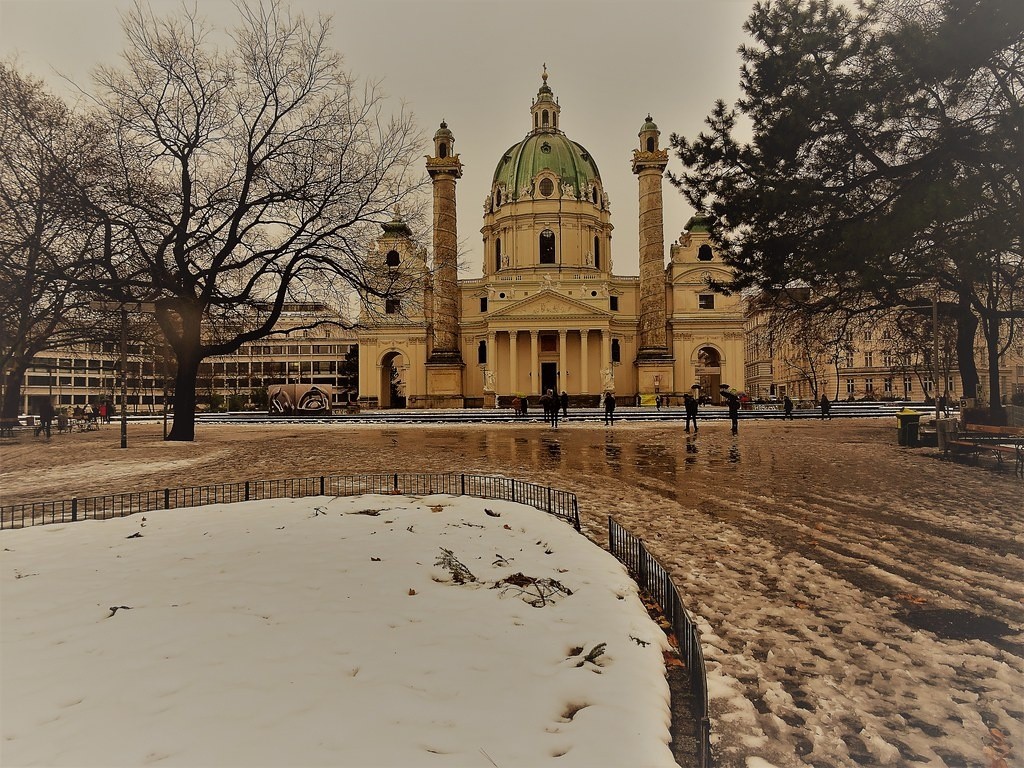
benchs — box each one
[0,420,98,441]
[944,430,1024,478]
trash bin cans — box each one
[959,394,976,420]
[58,413,68,430]
[896,408,921,446]
[27,417,34,426]
[938,397,946,411]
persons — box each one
[604,392,615,426]
[511,395,529,418]
[655,396,661,411]
[726,398,740,436]
[683,393,705,433]
[782,396,793,421]
[84,404,106,425]
[666,396,670,407]
[35,398,53,438]
[820,394,831,420]
[538,389,568,428]
[939,394,947,418]
[67,405,74,433]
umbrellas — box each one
[691,384,701,389]
[719,390,739,400]
[719,384,730,389]
